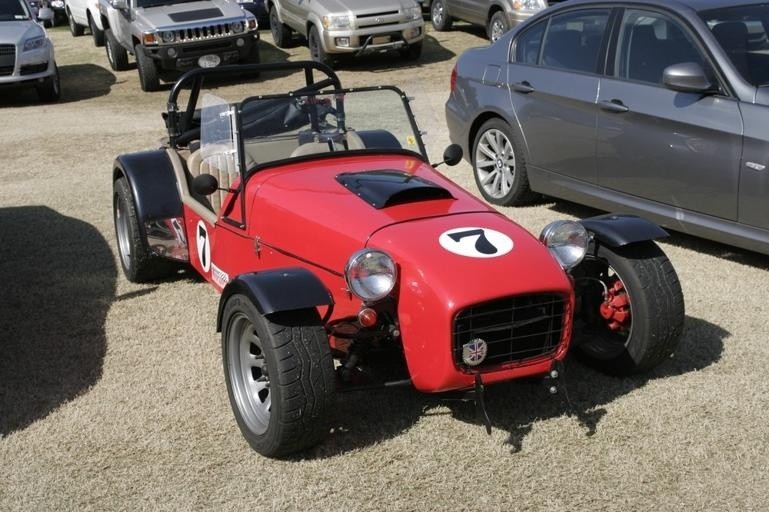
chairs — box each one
[525,20,752,86]
[185,141,348,219]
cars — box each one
[112,60,685,461]
[445,0,769,255]
